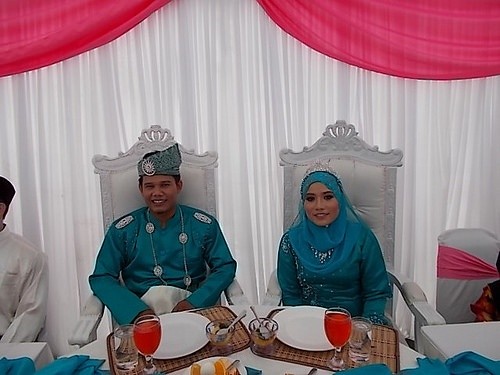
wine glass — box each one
[248,318,279,354]
[324,308,352,370]
[205,319,235,354]
[132,315,162,375]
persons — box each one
[88,143,237,332]
[0,176,49,342]
[470,251,500,323]
[277,158,393,325]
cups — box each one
[349,317,372,363]
[114,324,138,371]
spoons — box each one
[250,306,269,333]
[217,313,247,335]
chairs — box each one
[68,124,255,348]
[262,120,446,356]
[435,227,500,324]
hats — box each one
[138,143,183,177]
[0,176,16,219]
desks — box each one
[420,321,500,364]
[59,305,427,375]
[0,342,56,369]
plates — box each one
[273,306,335,351]
[187,357,247,375]
[152,312,215,360]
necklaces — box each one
[146,204,191,291]
[310,246,334,264]
